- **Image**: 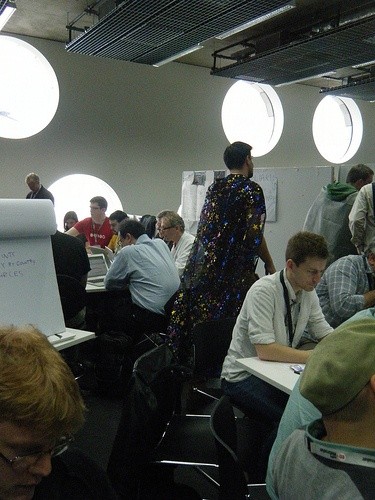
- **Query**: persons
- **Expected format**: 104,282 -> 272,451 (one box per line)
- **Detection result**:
104,218 -> 182,336
348,181 -> 375,255
64,211 -> 87,243
108,210 -> 130,253
219,233 -> 334,474
154,210 -> 177,251
0,323 -> 86,500
160,212 -> 197,278
298,242 -> 375,350
171,142 -> 276,380
65,196 -> 113,249
25,173 -> 55,205
266,308 -> 374,499
50,229 -> 91,329
303,164 -> 373,276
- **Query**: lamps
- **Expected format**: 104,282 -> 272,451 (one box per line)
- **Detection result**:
221,80 -> 284,158
312,94 -> 364,165
0,0 -> 17,30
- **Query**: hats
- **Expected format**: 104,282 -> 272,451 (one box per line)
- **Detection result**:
303,314 -> 375,415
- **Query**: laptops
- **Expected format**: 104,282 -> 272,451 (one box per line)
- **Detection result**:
86,246 -> 115,287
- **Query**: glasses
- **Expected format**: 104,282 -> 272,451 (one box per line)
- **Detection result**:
0,431 -> 74,474
159,225 -> 178,232
90,206 -> 101,211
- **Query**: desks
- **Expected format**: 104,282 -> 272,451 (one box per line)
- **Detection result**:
86,279 -> 108,329
48,328 -> 97,351
236,357 -> 305,395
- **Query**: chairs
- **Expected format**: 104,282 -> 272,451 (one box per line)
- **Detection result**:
150,387 -> 267,500
132,314 -> 165,373
